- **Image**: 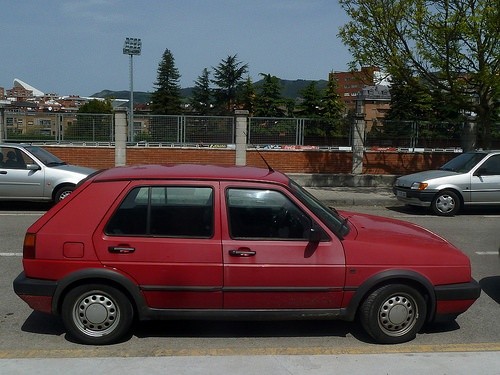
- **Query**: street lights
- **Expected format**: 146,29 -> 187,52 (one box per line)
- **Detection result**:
122,37 -> 143,143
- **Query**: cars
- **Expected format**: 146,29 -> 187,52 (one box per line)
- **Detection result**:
393,150 -> 499,216
14,132 -> 480,345
0,141 -> 100,204
